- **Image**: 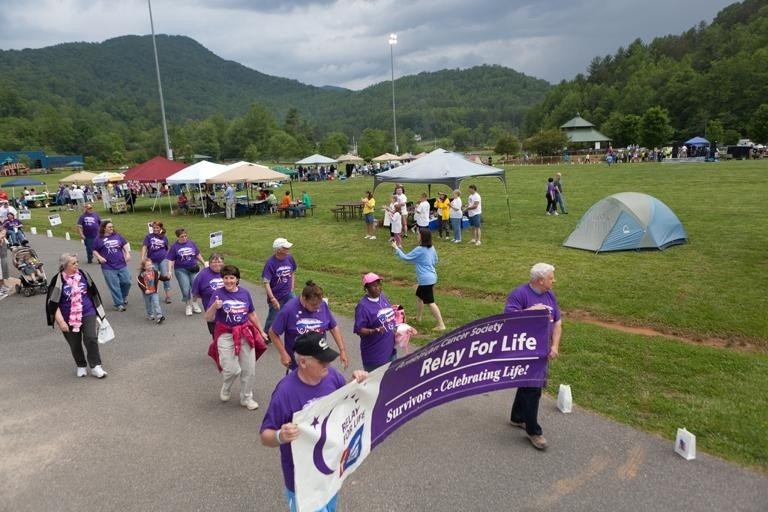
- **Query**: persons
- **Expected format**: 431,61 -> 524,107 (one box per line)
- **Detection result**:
263,237 -> 298,343
549,172 -> 568,214
361,185 -> 483,245
0,187 -> 50,299
267,279 -> 349,376
57,181 -> 146,211
544,177 -> 560,215
45,253 -> 116,378
298,160 -> 410,181
74,205 -> 270,410
585,153 -> 590,164
176,182 -> 313,221
606,143 -> 710,168
352,272 -> 419,373
390,229 -> 447,331
260,332 -> 368,512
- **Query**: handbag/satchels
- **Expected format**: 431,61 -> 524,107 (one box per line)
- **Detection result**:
557,383 -> 572,414
674,426 -> 698,461
94,315 -> 118,344
191,265 -> 200,274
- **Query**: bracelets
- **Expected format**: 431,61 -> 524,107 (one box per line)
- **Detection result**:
277,429 -> 283,445
270,296 -> 277,304
375,328 -> 381,336
369,328 -> 374,336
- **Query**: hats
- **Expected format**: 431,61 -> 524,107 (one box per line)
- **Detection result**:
273,237 -> 294,250
295,331 -> 339,364
360,271 -> 384,287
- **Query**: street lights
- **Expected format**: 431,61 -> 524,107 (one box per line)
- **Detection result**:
388,30 -> 398,153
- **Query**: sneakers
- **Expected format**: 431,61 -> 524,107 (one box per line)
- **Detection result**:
240,396 -> 259,411
114,297 -> 202,324
509,417 -> 548,450
219,384 -> 232,401
363,233 -> 482,251
77,364 -> 107,379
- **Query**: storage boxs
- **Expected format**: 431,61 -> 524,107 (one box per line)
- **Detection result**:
461,217 -> 472,230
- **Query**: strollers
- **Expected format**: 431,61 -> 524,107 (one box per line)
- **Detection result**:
4,240 -> 50,298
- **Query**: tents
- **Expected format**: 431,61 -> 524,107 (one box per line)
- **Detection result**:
562,192 -> 688,255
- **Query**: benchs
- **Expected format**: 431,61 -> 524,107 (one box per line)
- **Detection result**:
183,194 -> 442,230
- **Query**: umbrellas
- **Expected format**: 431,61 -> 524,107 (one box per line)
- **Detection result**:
687,137 -> 710,149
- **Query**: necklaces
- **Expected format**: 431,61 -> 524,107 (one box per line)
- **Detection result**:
505,263 -> 562,448
